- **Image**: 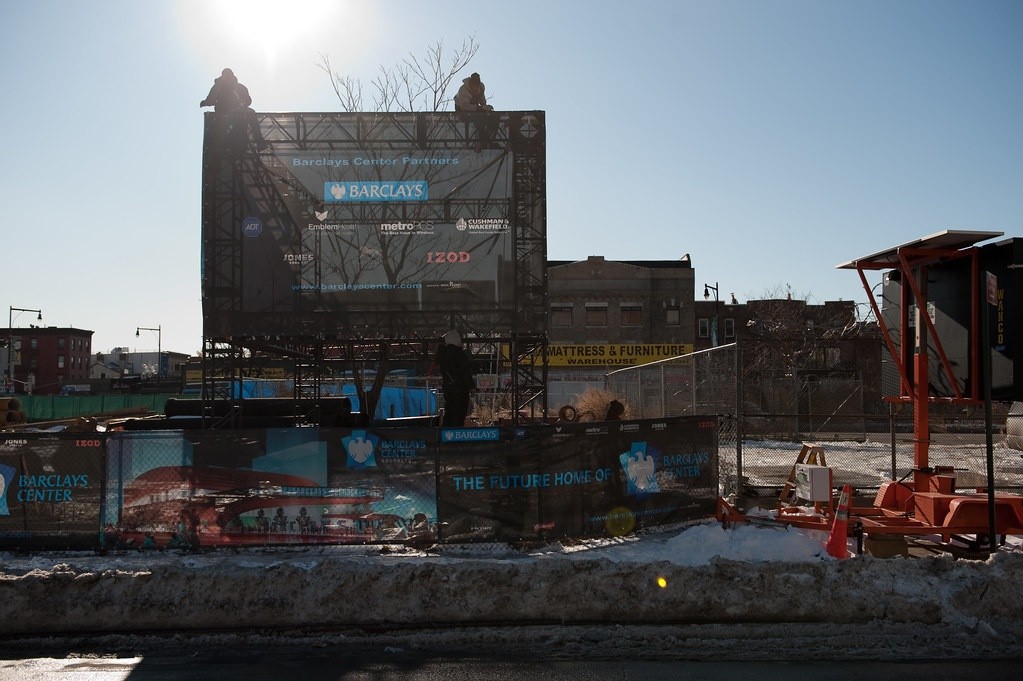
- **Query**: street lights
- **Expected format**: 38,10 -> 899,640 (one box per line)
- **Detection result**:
136,325 -> 161,380
8,306 -> 42,378
704,282 -> 720,346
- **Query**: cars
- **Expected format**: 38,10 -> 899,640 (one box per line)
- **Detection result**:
1005,402 -> 1023,452
741,401 -> 777,432
682,404 -> 751,433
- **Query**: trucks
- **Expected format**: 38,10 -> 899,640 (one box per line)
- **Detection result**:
62,384 -> 91,394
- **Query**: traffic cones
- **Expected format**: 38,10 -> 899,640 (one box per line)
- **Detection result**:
822,484 -> 852,558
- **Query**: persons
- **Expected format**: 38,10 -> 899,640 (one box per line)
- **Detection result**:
199,68 -> 270,152
453,73 -> 493,112
439,330 -> 476,426
605,401 -> 624,432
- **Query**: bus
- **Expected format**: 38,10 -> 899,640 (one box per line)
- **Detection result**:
134,382 -> 160,394
